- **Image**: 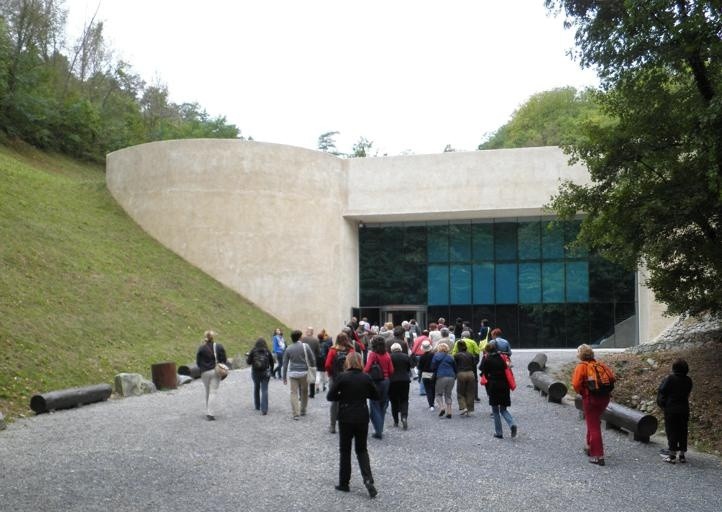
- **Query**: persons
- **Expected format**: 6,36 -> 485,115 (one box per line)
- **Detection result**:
656,357 -> 693,464
573,344 -> 616,466
326,351 -> 382,497
197,330 -> 228,419
246,314 -> 518,439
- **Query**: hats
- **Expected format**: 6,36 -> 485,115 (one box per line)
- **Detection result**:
421,340 -> 432,352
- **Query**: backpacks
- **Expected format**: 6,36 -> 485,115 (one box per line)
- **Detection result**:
254,353 -> 269,370
580,360 -> 614,394
332,351 -> 347,378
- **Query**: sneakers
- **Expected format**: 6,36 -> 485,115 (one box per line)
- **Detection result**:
335,485 -> 350,492
206,415 -> 215,420
663,455 -> 686,465
395,420 -> 407,429
430,407 -> 469,418
372,432 -> 381,439
255,408 -> 268,415
294,410 -> 306,420
494,424 -> 517,439
365,480 -> 377,498
584,449 -> 606,466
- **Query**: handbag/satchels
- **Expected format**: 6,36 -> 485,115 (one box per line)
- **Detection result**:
214,362 -> 229,380
504,366 -> 516,391
368,363 -> 385,381
422,371 -> 434,380
308,366 -> 317,383
